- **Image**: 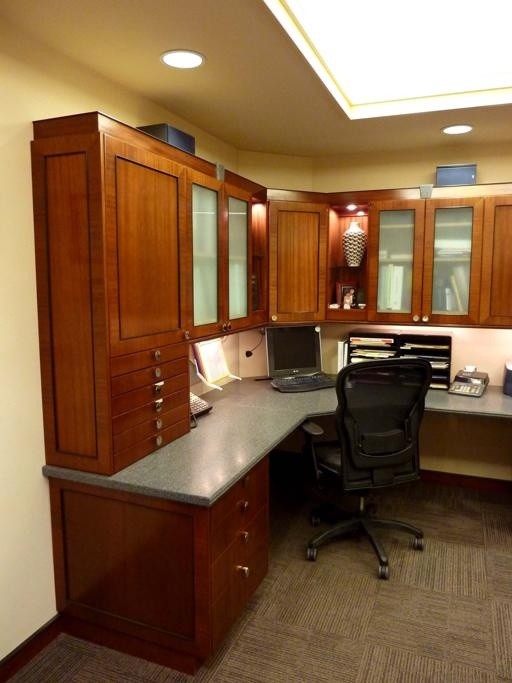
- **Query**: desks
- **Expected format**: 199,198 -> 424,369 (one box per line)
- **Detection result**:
42,381 -> 512,677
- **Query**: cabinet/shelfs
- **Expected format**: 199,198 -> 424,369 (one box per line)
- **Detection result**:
30,110 -> 512,477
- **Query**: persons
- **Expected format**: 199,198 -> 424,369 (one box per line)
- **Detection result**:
349,288 -> 355,296
344,292 -> 352,303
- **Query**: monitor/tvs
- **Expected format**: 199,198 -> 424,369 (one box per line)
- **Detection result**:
265,325 -> 322,378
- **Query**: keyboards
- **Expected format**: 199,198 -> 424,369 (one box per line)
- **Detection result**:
272,375 -> 337,393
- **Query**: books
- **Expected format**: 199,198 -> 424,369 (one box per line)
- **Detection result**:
350,336 -> 448,388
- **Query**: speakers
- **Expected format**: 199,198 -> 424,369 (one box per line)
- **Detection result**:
132,123 -> 196,155
436,164 -> 476,187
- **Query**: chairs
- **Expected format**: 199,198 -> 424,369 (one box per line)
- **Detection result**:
299,358 -> 433,579
